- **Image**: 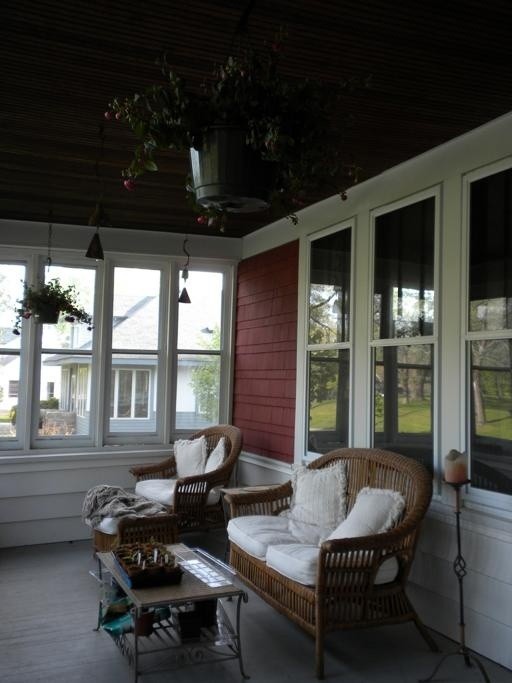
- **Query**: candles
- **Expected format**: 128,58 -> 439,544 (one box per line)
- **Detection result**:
444,450 -> 469,482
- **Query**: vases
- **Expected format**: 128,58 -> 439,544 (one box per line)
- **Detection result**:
33,310 -> 60,324
190,126 -> 272,213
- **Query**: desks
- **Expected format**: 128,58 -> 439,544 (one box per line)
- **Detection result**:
93,543 -> 251,682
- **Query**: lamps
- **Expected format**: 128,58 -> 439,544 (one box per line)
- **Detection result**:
178,239 -> 191,304
84,218 -> 104,261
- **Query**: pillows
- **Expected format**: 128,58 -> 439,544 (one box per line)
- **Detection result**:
288,463 -> 347,544
205,436 -> 227,474
173,435 -> 209,478
318,488 -> 406,557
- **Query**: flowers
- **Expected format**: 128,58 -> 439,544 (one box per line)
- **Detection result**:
12,278 -> 95,335
102,42 -> 356,228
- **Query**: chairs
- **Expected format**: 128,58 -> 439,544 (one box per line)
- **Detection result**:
88,514 -> 179,585
225,447 -> 438,677
129,425 -> 242,532
